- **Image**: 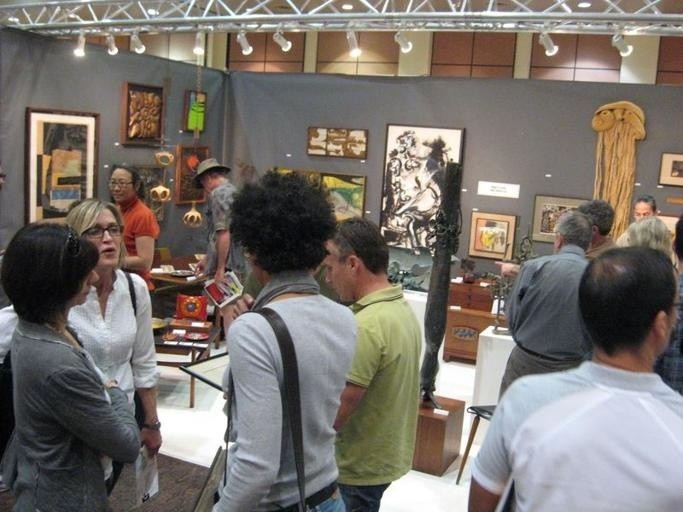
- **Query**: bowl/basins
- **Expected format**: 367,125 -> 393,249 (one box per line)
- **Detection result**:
152,318 -> 168,336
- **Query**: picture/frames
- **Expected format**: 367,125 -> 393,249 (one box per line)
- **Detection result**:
184,89 -> 208,133
659,153 -> 683,186
307,127 -> 368,158
176,143 -> 208,206
467,211 -> 517,262
24,105 -> 101,230
532,194 -> 590,243
119,79 -> 163,148
378,123 -> 465,252
131,166 -> 169,223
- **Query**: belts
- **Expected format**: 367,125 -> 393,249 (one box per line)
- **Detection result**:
277,480 -> 338,512
517,342 -> 558,362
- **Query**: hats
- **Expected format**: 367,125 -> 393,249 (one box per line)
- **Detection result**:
192,158 -> 230,178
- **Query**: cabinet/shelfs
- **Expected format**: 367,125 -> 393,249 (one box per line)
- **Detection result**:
443,306 -> 507,363
472,326 -> 515,408
447,277 -> 495,313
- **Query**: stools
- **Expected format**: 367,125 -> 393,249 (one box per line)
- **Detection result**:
456,403 -> 497,483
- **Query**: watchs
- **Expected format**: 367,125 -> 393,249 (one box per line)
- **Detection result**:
142,422 -> 161,429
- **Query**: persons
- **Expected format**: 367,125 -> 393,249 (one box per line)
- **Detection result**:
0,198 -> 162,512
191,157 -> 250,339
0,222 -> 141,512
499,210 -> 593,406
633,194 -> 656,219
231,256 -> 351,319
109,164 -> 160,296
628,214 -> 673,263
654,214 -> 683,399
495,199 -> 620,278
325,216 -> 421,512
468,247 -> 683,511
212,168 -> 359,512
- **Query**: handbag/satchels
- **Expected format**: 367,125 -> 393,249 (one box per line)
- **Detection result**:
0,349 -> 15,463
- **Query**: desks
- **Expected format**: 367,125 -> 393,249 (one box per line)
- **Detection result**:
148,254 -> 225,349
155,321 -> 221,407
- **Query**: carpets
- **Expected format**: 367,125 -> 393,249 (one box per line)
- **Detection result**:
1,454 -> 210,511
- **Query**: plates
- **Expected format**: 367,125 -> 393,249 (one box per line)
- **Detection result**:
170,270 -> 193,278
150,274 -> 168,276
185,332 -> 209,342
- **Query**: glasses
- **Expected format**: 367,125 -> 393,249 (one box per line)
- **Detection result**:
85,224 -> 124,237
672,296 -> 683,310
108,181 -> 134,187
58,224 -> 80,271
